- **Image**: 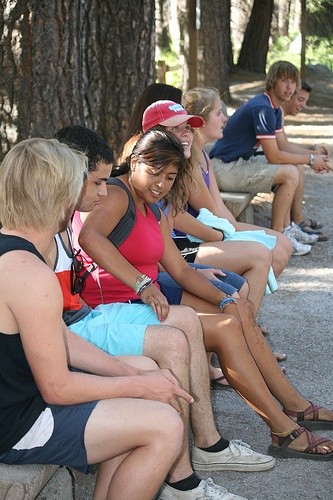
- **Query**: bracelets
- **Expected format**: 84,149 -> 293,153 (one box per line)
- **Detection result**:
308,154 -> 315,167
311,143 -> 316,150
132,274 -> 153,295
218,295 -> 237,312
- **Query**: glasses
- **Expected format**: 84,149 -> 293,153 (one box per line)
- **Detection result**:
69,248 -> 85,296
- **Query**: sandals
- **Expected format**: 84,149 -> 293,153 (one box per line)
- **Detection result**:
283,401 -> 333,427
295,223 -> 330,241
268,428 -> 333,460
302,218 -> 322,229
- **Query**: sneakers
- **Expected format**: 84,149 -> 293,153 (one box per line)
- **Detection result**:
279,221 -> 319,244
192,440 -> 277,472
158,477 -> 250,499
278,232 -> 311,256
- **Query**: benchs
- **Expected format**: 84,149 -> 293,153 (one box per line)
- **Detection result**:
0,463 -> 77,500
220,190 -> 256,226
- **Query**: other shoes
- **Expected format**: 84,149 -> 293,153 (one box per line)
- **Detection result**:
209,372 -> 238,389
274,352 -> 287,361
259,324 -> 270,336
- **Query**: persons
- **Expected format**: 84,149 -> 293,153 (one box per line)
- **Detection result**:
64,130 -> 333,463
20,124 -> 276,500
249,78 -> 329,241
115,83 -> 182,170
205,60 -> 333,256
103,166 -> 256,390
122,100 -> 288,361
182,83 -> 293,329
0,137 -> 194,500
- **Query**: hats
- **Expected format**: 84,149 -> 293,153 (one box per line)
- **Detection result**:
142,100 -> 204,133
